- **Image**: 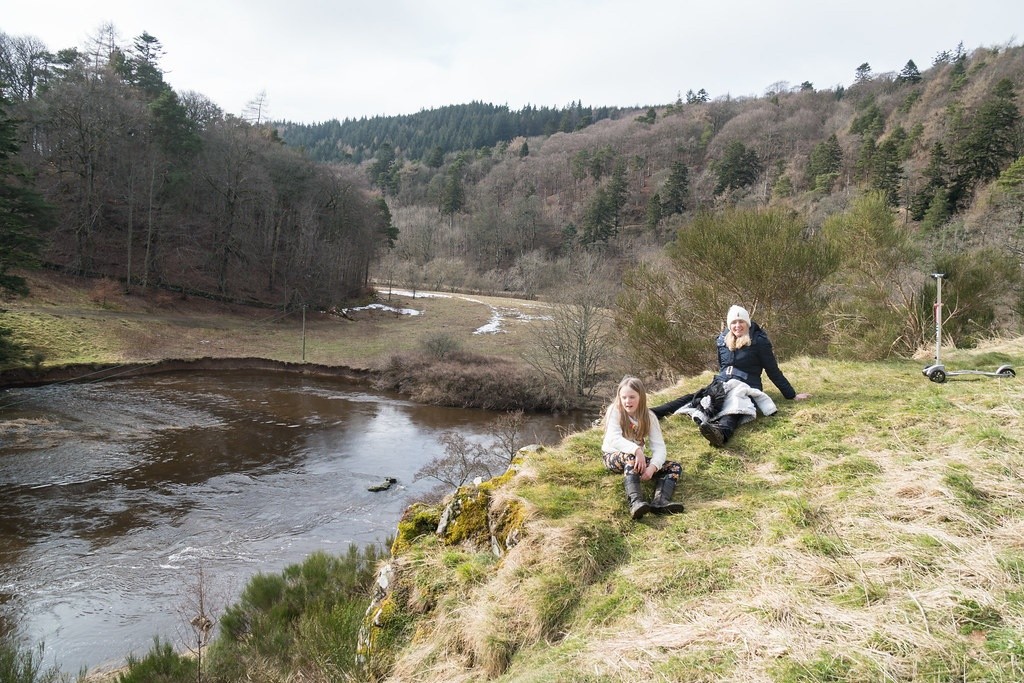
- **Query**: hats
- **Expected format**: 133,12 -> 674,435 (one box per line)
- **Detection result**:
727,304 -> 751,331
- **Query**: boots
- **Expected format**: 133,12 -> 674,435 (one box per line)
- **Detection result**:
622,475 -> 649,520
649,478 -> 684,515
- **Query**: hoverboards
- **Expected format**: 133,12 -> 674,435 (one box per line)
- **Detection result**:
921,273 -> 1016,383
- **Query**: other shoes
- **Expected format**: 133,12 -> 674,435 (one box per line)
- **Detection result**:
699,421 -> 725,448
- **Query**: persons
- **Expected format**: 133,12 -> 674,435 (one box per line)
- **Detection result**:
601,377 -> 684,521
648,304 -> 814,448
